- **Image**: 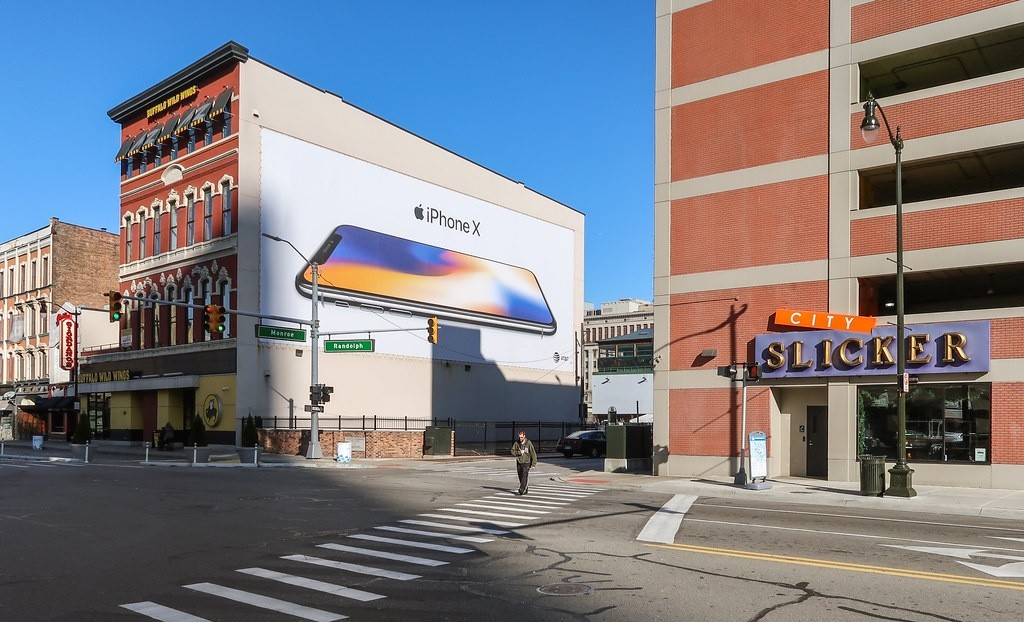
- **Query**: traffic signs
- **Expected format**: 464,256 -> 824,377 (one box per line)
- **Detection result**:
310,405 -> 324,413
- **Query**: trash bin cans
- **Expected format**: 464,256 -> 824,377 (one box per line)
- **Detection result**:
335,443 -> 352,462
32,435 -> 44,450
857,455 -> 887,496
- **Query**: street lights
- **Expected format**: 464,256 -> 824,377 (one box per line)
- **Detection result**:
38,300 -> 83,436
859,92 -> 919,500
260,231 -> 324,459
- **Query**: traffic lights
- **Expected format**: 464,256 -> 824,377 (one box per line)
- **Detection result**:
746,364 -> 762,379
213,305 -> 226,333
724,365 -> 737,376
309,386 -> 320,401
321,386 -> 333,403
427,317 -> 437,345
202,304 -> 213,333
109,289 -> 122,323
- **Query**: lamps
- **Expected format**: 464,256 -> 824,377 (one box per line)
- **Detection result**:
701,349 -> 717,357
264,370 -> 270,377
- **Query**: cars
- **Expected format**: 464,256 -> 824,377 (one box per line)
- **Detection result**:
554,429 -> 606,459
863,424 -> 977,461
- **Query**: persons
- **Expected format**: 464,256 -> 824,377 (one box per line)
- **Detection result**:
158,422 -> 175,451
511,431 -> 538,495
